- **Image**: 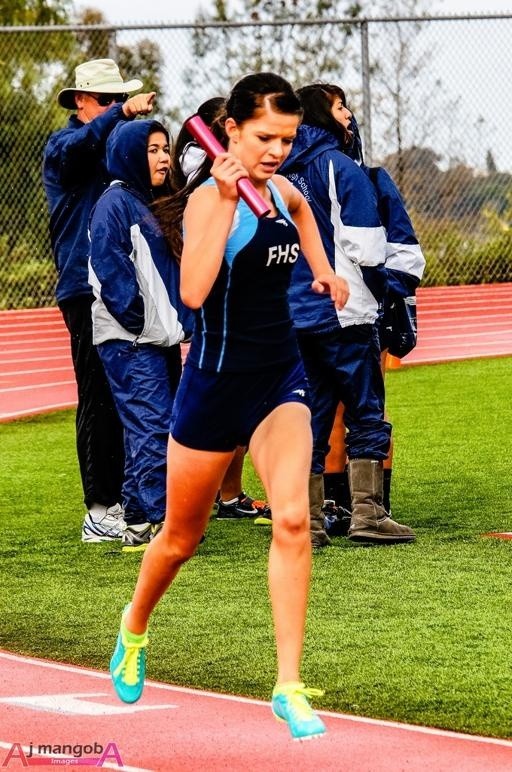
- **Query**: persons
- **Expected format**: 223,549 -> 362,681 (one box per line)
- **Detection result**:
41,55 -> 430,547
106,72 -> 351,744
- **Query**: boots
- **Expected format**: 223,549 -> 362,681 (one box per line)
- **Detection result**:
309,457 -> 418,550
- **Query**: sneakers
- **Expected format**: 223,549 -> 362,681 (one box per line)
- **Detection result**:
271,684 -> 326,741
121,524 -> 161,553
108,603 -> 149,703
215,494 -> 267,518
79,506 -> 124,540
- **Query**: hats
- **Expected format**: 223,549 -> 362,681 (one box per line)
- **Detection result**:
58,59 -> 144,103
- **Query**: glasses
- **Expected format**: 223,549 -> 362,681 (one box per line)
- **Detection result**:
82,91 -> 128,106
